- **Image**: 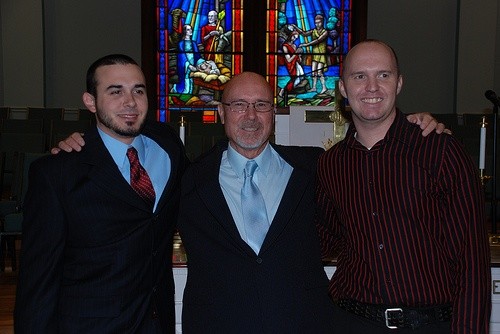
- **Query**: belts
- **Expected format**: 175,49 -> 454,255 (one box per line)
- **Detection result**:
333,293 -> 453,330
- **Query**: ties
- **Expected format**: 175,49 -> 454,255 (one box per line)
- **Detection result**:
241,160 -> 271,257
126,147 -> 156,214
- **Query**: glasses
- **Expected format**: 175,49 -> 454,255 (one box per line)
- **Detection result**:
222,100 -> 275,113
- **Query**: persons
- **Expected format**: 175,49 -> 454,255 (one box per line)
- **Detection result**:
49,72 -> 452,334
316,39 -> 491,334
13,54 -> 190,334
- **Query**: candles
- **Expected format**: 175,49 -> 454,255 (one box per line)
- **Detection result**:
479,116 -> 488,170
179,115 -> 184,146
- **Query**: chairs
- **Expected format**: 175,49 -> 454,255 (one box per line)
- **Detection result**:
0,107 -> 97,274
156,112 -> 226,162
435,113 -> 500,221
